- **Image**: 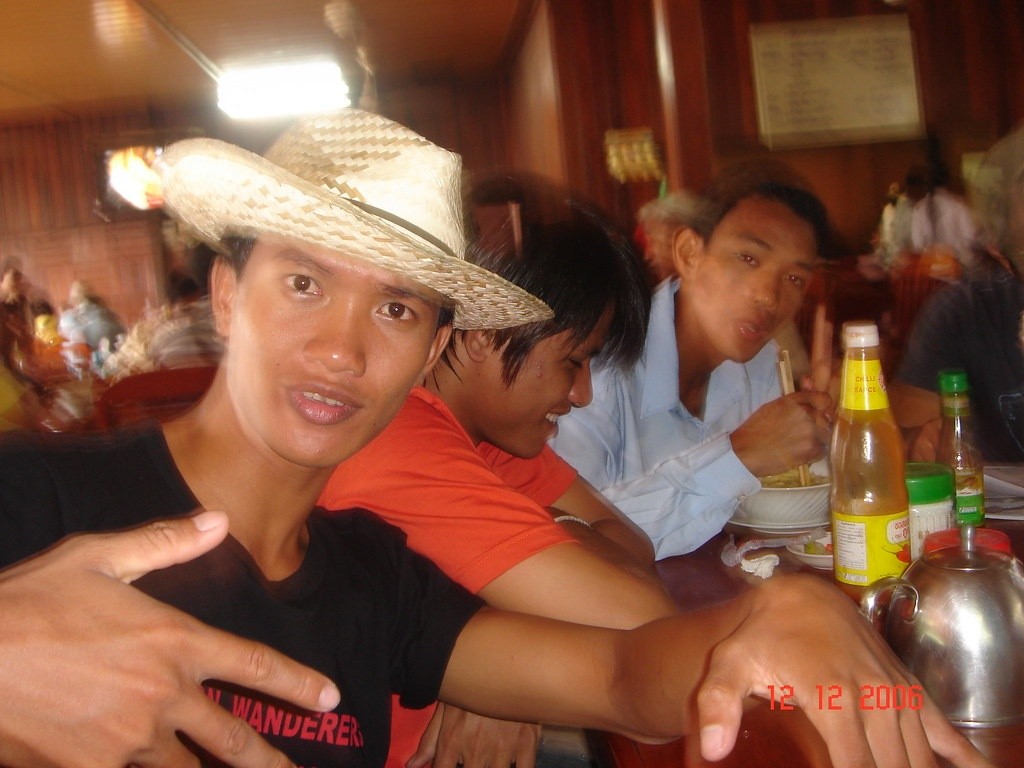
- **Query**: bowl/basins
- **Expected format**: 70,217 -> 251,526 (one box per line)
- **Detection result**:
739,457 -> 833,524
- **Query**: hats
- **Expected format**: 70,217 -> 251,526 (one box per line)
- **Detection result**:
159,108 -> 558,330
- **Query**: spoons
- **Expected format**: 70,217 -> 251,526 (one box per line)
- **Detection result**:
986,505 -> 1024,513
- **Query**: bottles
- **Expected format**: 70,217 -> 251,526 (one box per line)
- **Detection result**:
937,372 -> 985,528
830,322 -> 911,605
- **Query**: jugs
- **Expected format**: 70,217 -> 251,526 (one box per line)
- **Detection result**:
860,527 -> 1024,728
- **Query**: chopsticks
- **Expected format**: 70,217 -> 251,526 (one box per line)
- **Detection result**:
779,350 -> 812,486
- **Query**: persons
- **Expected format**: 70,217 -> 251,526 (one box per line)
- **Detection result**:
0,135 -> 1024,768
0,109 -> 1001,768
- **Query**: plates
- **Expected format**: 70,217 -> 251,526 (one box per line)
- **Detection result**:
787,532 -> 834,570
728,517 -> 831,535
984,472 -> 1024,521
